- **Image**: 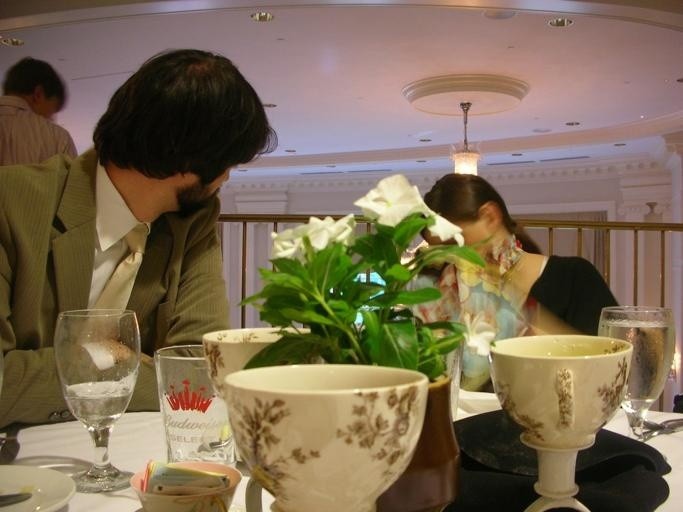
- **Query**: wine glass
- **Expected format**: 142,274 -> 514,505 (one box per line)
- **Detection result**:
599,306 -> 676,443
487,332 -> 633,512
52,307 -> 142,494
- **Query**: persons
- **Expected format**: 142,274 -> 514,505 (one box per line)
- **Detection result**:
0,50 -> 279,441
1,53 -> 79,165
417,171 -> 656,400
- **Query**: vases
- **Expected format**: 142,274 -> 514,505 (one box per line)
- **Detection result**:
375,375 -> 464,511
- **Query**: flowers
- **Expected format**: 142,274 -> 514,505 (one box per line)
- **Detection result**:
231,175 -> 493,383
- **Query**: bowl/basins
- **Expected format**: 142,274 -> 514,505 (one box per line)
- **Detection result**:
130,460 -> 242,512
202,327 -> 311,398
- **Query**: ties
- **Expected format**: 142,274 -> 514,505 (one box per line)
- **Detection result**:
85,224 -> 147,317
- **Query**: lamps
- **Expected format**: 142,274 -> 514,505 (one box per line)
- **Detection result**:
451,101 -> 481,175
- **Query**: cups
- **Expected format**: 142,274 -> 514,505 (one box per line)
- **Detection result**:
224,362 -> 428,512
154,344 -> 240,464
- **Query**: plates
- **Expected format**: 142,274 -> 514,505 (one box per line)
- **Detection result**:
0,465 -> 77,510
